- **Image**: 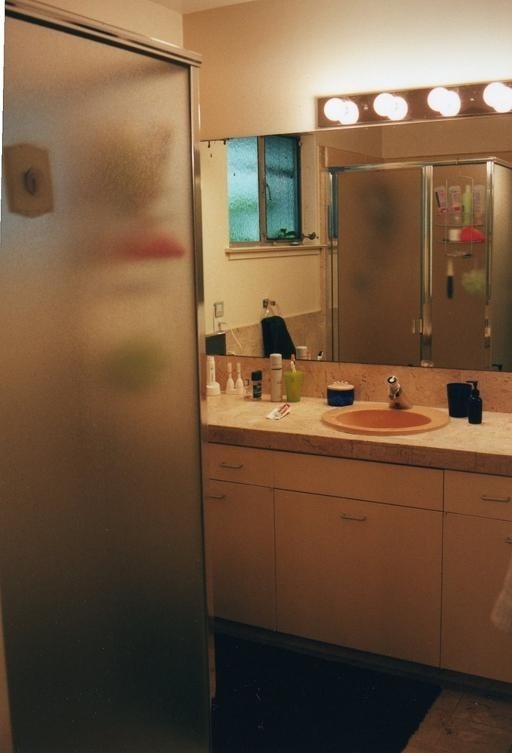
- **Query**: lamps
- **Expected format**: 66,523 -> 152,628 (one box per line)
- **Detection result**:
374,94 -> 407,121
324,97 -> 359,125
428,87 -> 460,117
484,82 -> 512,113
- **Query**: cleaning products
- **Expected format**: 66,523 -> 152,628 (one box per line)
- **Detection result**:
434,186 -> 449,223
449,185 -> 462,224
462,185 -> 472,224
473,184 -> 486,224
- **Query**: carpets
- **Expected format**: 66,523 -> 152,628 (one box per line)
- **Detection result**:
209,634 -> 443,753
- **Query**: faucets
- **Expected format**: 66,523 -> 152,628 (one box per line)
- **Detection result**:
387,375 -> 413,410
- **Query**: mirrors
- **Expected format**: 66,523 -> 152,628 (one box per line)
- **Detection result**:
199,114 -> 512,372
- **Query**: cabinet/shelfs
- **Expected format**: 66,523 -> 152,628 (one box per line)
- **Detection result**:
328,156 -> 512,372
205,442 -> 512,687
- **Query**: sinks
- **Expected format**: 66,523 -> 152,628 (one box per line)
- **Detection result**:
321,404 -> 450,436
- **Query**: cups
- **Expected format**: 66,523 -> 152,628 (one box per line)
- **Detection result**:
447,383 -> 471,418
283,371 -> 304,402
448,229 -> 461,241
